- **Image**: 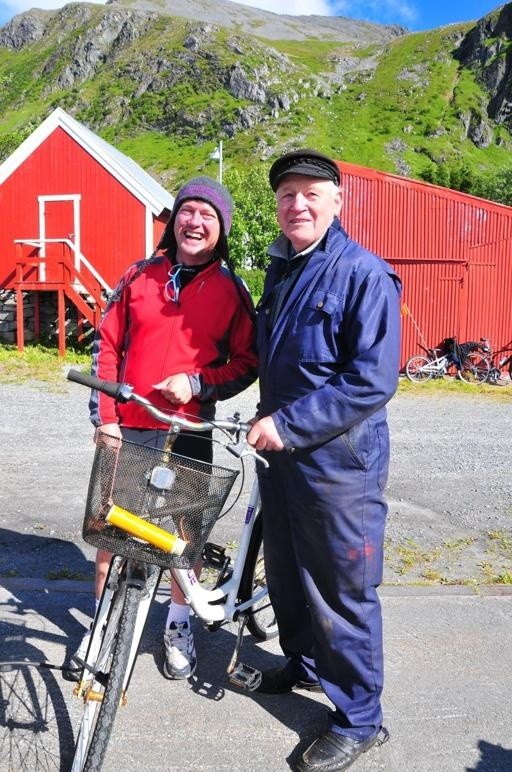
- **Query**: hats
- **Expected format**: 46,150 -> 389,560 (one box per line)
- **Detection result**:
269,150 -> 340,193
158,175 -> 234,260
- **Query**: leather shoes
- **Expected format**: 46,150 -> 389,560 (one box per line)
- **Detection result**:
257,659 -> 325,695
295,730 -> 378,772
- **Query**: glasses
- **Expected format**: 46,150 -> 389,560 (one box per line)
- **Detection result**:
165,263 -> 183,303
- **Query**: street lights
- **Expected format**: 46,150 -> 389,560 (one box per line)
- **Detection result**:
211,140 -> 224,185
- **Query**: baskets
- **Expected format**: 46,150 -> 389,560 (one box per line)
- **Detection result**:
82,432 -> 242,570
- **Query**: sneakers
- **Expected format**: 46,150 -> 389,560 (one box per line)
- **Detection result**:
163,620 -> 198,680
66,621 -> 109,683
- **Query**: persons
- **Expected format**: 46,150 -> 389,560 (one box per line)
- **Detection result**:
245,149 -> 402,772
66,177 -> 256,686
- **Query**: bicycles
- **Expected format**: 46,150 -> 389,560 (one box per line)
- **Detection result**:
458,337 -> 512,387
405,335 -> 491,385
66,366 -> 281,771
